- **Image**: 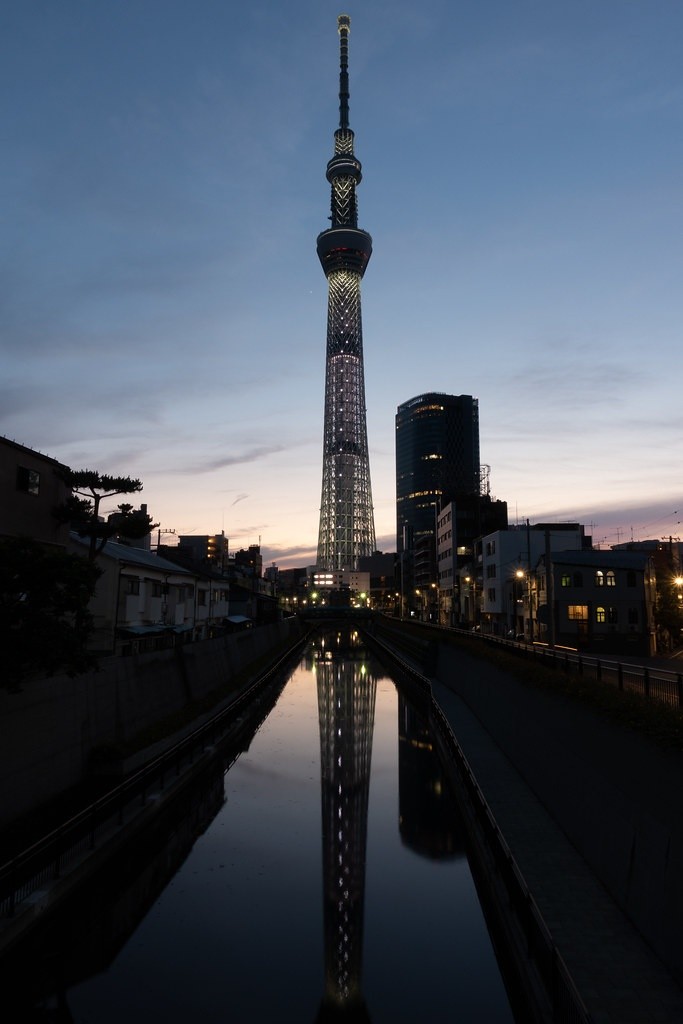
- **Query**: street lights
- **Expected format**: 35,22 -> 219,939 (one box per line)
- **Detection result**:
516,569 -> 534,644
465,577 -> 476,631
416,590 -> 423,622
432,584 -> 442,625
